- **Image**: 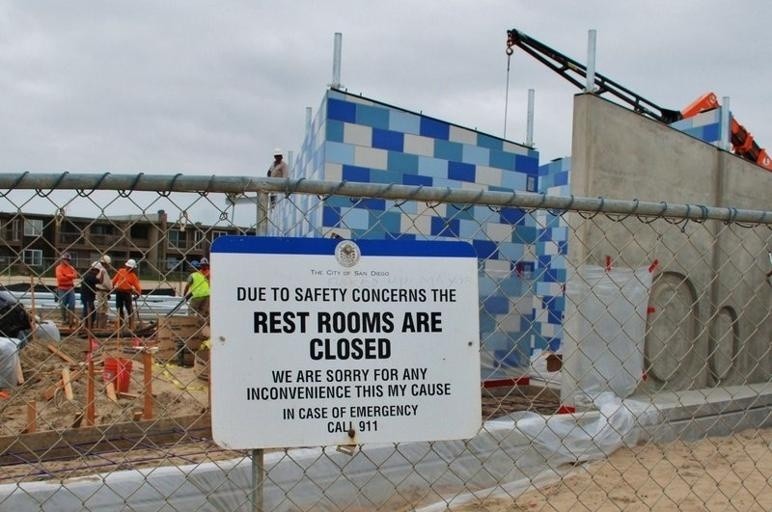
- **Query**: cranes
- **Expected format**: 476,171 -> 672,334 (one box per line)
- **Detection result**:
504,27 -> 772,169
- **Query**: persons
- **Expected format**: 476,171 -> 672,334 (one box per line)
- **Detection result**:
80,261 -> 106,329
183,260 -> 210,318
200,257 -> 210,287
56,252 -> 79,326
266,149 -> 288,208
92,254 -> 112,329
111,258 -> 141,330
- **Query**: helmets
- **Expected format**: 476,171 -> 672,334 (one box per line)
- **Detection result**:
63,253 -> 71,259
192,258 -> 209,267
125,259 -> 137,268
92,261 -> 101,269
101,255 -> 111,263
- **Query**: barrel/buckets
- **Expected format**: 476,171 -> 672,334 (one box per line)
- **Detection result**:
103,355 -> 132,394
194,351 -> 210,380
